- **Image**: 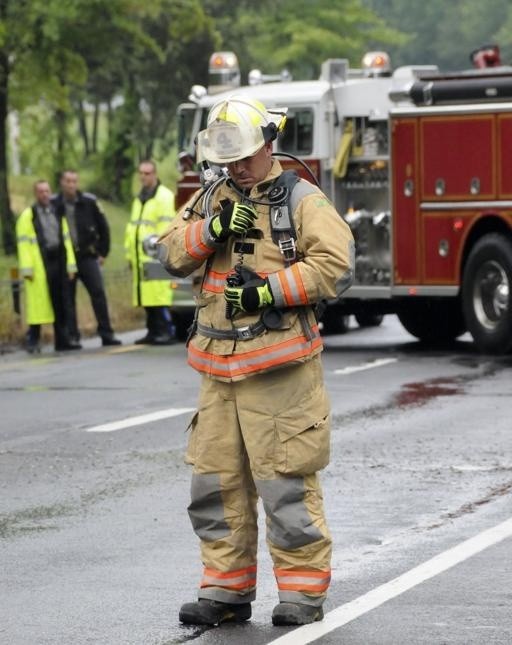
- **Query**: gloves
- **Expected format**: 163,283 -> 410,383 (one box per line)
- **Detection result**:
207,201 -> 259,244
222,264 -> 274,315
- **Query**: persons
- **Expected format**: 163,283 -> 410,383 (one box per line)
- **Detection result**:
15,169 -> 121,355
124,161 -> 176,345
156,97 -> 355,624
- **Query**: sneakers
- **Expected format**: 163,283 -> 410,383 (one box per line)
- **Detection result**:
177,598 -> 253,627
24,329 -> 177,358
270,601 -> 325,626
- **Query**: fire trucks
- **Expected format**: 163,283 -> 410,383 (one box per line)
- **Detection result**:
143,50 -> 511,349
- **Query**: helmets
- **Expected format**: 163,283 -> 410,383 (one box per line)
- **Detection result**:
200,92 -> 289,164
